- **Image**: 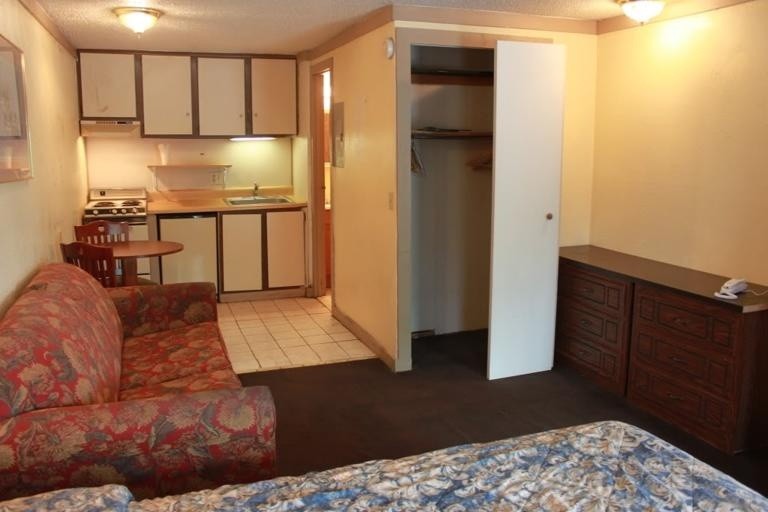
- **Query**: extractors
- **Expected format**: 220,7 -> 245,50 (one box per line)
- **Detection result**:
79,119 -> 140,138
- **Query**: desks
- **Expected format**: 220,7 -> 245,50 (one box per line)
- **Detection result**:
79,240 -> 184,285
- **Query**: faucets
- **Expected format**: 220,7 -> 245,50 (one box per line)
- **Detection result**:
252,183 -> 260,197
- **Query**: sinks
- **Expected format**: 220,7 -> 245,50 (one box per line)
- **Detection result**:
222,195 -> 293,206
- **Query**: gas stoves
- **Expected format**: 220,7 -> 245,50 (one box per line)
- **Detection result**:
84,199 -> 147,217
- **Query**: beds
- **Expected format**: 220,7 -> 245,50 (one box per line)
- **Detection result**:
0,417 -> 767,511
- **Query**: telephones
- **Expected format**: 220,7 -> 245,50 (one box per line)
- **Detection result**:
720,278 -> 748,295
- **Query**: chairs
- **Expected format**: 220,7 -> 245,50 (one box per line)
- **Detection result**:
74,220 -> 129,277
59,241 -> 117,287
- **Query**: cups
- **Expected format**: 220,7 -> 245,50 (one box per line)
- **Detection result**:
157,142 -> 170,166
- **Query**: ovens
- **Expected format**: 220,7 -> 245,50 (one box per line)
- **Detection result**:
83,218 -> 151,281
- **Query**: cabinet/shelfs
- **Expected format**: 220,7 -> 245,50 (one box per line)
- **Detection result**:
556,262 -> 632,404
158,216 -> 218,295
79,51 -> 137,119
613,0 -> 670,25
141,54 -> 194,135
628,282 -> 767,454
221,209 -> 305,292
197,56 -> 298,136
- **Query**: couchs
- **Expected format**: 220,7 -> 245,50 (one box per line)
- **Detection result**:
0,262 -> 279,502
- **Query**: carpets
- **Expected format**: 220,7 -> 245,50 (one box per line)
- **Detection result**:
216,296 -> 379,374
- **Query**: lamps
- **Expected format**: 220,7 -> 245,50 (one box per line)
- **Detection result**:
112,7 -> 161,33
